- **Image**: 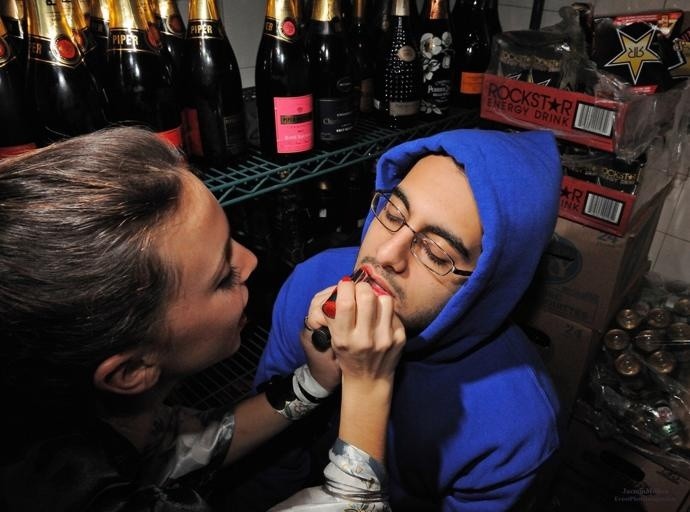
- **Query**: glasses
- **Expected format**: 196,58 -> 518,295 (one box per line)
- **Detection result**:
370,189 -> 473,276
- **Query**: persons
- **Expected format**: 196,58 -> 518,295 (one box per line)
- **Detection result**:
0,119 -> 407,512
246,128 -> 566,512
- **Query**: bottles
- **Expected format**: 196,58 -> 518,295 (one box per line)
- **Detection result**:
0,0 -> 488,171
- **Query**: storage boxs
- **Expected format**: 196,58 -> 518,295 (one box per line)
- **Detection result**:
478,71 -> 689,151
537,400 -> 688,511
478,121 -> 680,419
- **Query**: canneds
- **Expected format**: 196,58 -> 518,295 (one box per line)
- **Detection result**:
586,281 -> 690,460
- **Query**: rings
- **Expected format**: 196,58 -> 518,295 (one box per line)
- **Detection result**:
303,315 -> 314,332
